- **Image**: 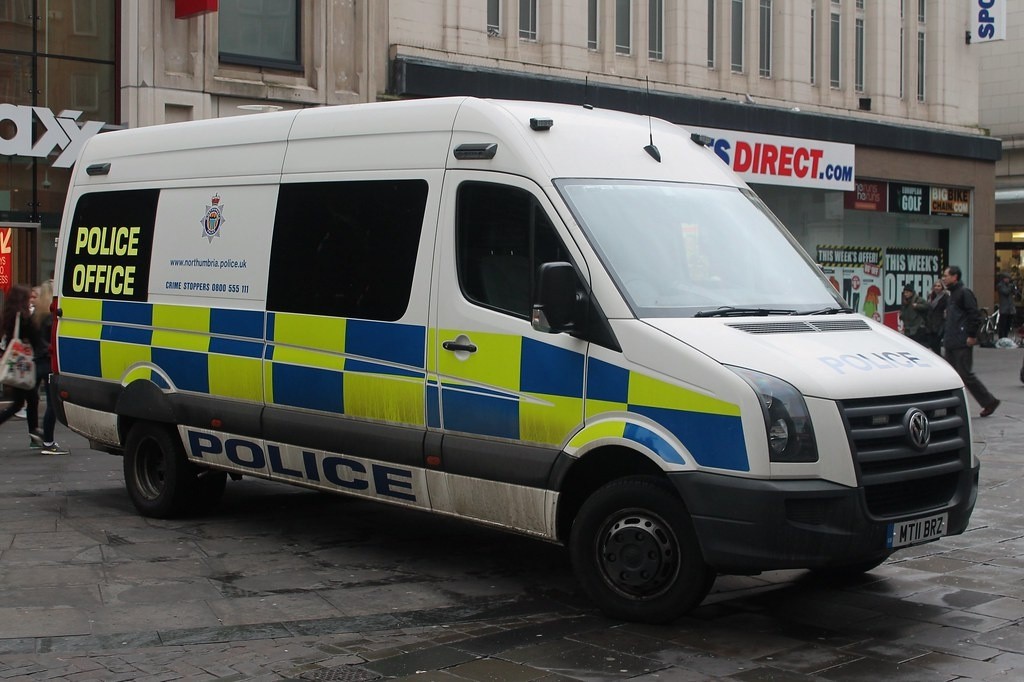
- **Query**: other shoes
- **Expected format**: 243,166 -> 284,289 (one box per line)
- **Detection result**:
30,443 -> 43,449
980,399 -> 1000,417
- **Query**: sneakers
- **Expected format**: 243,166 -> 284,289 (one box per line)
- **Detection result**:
41,442 -> 69,454
28,429 -> 43,439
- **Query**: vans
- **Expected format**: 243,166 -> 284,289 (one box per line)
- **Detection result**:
47,96 -> 983,626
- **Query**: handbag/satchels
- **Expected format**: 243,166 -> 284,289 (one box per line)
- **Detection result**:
0,312 -> 37,390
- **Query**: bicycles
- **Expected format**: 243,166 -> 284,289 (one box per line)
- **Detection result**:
979,303 -> 1015,344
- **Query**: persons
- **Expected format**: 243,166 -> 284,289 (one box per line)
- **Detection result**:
927,278 -> 950,354
997,273 -> 1017,340
0,278 -> 70,454
899,283 -> 927,347
942,265 -> 1001,417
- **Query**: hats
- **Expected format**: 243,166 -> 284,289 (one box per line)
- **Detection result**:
904,284 -> 914,292
1002,273 -> 1009,277
